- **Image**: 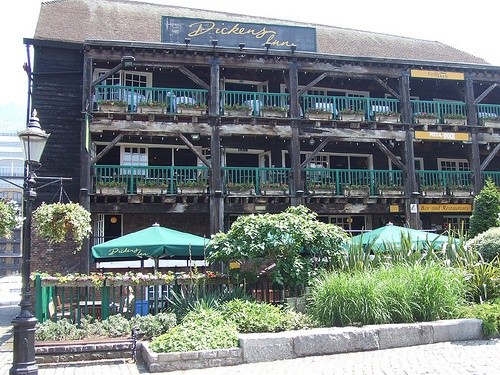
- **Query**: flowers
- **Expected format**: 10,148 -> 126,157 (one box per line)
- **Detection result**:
98,100 -> 500,123
413,112 -> 441,120
31,270 -> 228,288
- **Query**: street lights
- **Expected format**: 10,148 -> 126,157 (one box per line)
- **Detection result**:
9,107 -> 49,374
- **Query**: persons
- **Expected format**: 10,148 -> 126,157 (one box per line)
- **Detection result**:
117,286 -> 134,316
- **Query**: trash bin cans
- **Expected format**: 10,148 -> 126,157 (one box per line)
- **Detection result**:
133,300 -> 149,317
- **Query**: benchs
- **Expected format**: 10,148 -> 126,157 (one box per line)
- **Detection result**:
48,296 -> 134,323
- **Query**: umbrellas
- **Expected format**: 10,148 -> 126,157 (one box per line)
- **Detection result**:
341,222 -> 465,255
92,222 -> 214,313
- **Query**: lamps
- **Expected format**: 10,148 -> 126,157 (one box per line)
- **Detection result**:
290,45 -> 296,53
192,133 -> 201,139
185,38 -> 192,47
264,43 -> 271,52
239,43 -> 245,50
211,40 -> 219,48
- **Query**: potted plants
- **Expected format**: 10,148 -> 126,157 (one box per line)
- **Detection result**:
31,201 -> 94,254
96,179 -> 475,198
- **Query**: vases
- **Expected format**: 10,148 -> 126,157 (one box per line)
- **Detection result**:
106,280 -> 135,286
41,280 -> 95,288
140,279 -> 166,287
176,279 -> 199,285
206,278 -> 228,286
415,118 -> 439,126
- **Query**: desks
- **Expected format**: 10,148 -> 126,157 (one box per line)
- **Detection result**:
79,300 -> 101,317
